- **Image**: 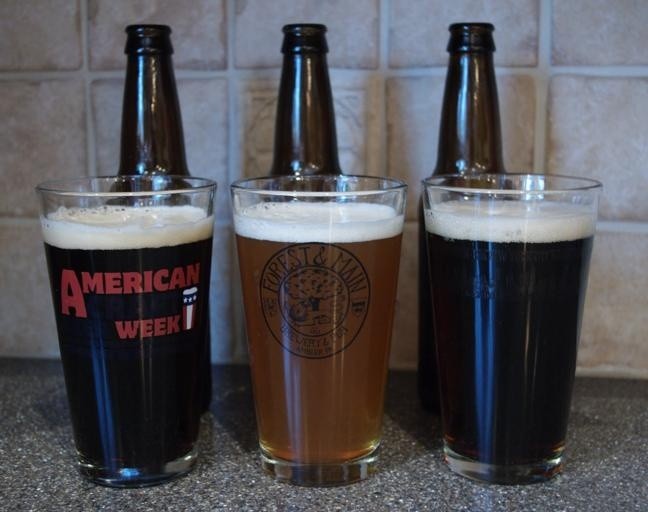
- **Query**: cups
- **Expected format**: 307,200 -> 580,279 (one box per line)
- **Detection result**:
36,175 -> 217,489
230,174 -> 409,487
420,173 -> 603,485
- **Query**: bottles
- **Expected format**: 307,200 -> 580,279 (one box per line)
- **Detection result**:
105,23 -> 196,208
264,23 -> 354,206
416,25 -> 519,414
